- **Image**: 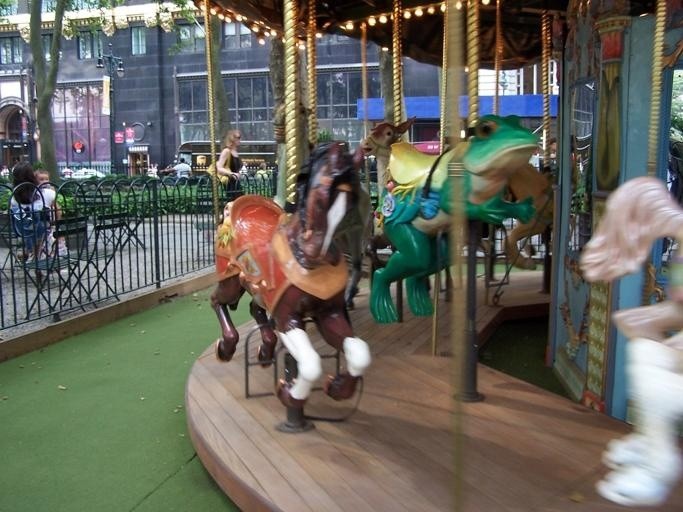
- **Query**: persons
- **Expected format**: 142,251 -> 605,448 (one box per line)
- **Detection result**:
548,137 -> 557,157
216,129 -> 247,201
256,162 -> 268,179
6,161 -> 56,262
163,158 -> 192,178
27,166 -> 63,225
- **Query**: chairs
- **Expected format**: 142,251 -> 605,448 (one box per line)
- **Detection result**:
194,197 -> 232,262
19,212 -> 129,323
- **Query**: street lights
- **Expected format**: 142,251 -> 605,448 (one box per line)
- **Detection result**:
95,43 -> 124,173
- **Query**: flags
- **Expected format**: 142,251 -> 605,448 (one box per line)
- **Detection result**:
101,74 -> 110,118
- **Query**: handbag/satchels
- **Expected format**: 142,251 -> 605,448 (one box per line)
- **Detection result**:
204,160 -> 232,186
